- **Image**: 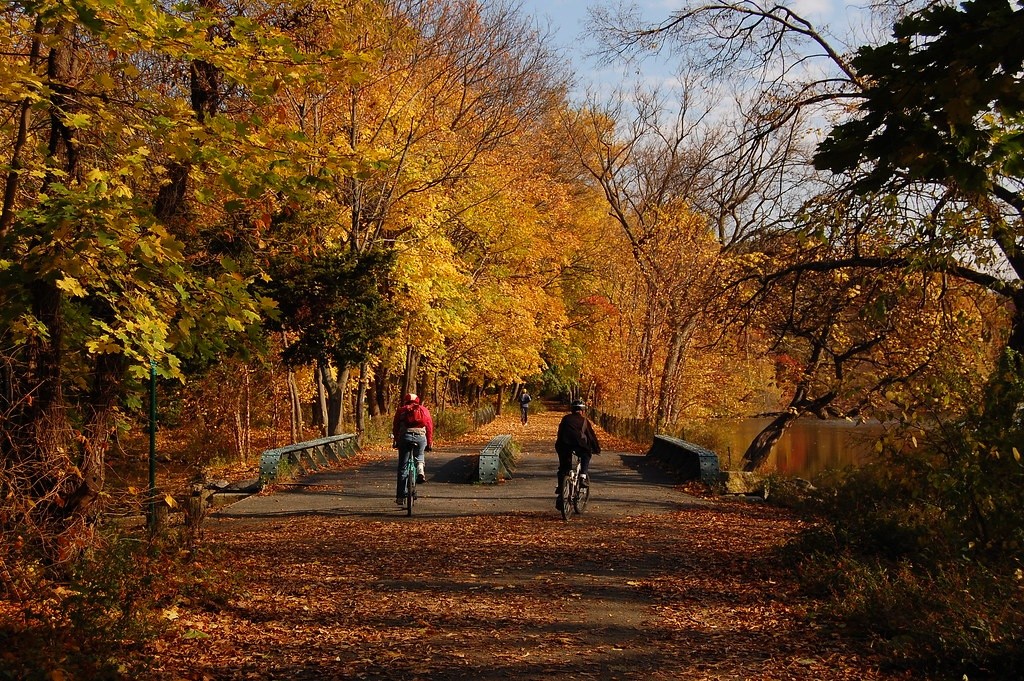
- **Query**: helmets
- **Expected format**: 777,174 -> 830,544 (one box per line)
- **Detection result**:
569,400 -> 586,411
404,393 -> 420,405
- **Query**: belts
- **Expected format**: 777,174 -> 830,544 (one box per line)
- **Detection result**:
406,432 -> 427,435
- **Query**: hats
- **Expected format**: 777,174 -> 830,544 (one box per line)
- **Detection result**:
522,389 -> 527,393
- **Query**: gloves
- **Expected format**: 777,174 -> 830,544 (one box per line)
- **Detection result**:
393,443 -> 398,450
424,445 -> 433,452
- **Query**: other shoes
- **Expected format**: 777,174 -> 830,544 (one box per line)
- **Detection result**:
415,471 -> 425,484
555,486 -> 567,494
397,498 -> 405,508
579,477 -> 590,488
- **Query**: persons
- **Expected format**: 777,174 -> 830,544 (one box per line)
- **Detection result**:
518,388 -> 531,426
555,401 -> 601,511
392,393 -> 432,505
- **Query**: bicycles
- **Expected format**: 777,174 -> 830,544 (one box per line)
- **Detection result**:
392,443 -> 432,517
557,450 -> 603,520
